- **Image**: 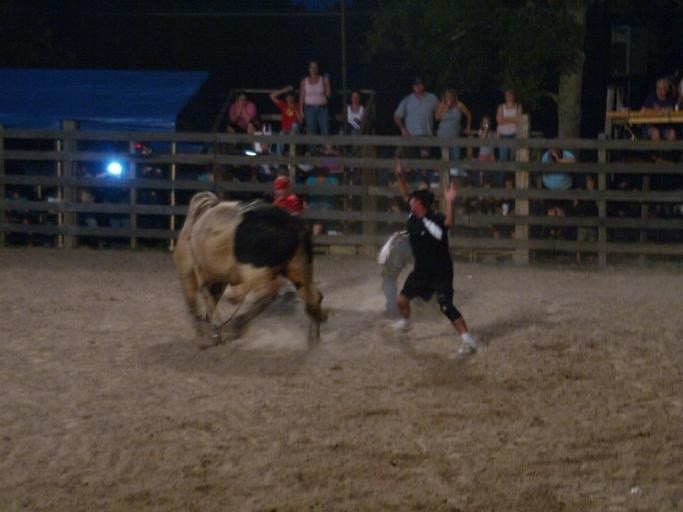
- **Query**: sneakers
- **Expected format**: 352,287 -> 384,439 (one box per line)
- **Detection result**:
458,339 -> 478,357
397,319 -> 414,334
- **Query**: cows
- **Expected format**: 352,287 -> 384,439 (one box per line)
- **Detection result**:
171,189 -> 329,346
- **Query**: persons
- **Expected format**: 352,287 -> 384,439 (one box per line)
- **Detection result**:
298,59 -> 333,152
269,174 -> 305,218
335,88 -> 368,172
296,145 -> 344,235
269,85 -> 302,170
132,141 -> 171,199
475,114 -> 495,186
392,155 -> 477,356
494,85 -> 521,189
434,87 -> 471,161
223,92 -> 259,153
392,76 -> 440,182
80,165 -> 113,226
639,75 -> 678,164
569,175 -> 599,263
536,137 -> 576,239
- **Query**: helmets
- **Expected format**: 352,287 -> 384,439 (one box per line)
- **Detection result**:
273,176 -> 292,190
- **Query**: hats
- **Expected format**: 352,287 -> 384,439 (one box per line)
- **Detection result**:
411,188 -> 436,203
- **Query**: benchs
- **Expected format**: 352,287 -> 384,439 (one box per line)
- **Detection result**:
213,86 -> 682,260
